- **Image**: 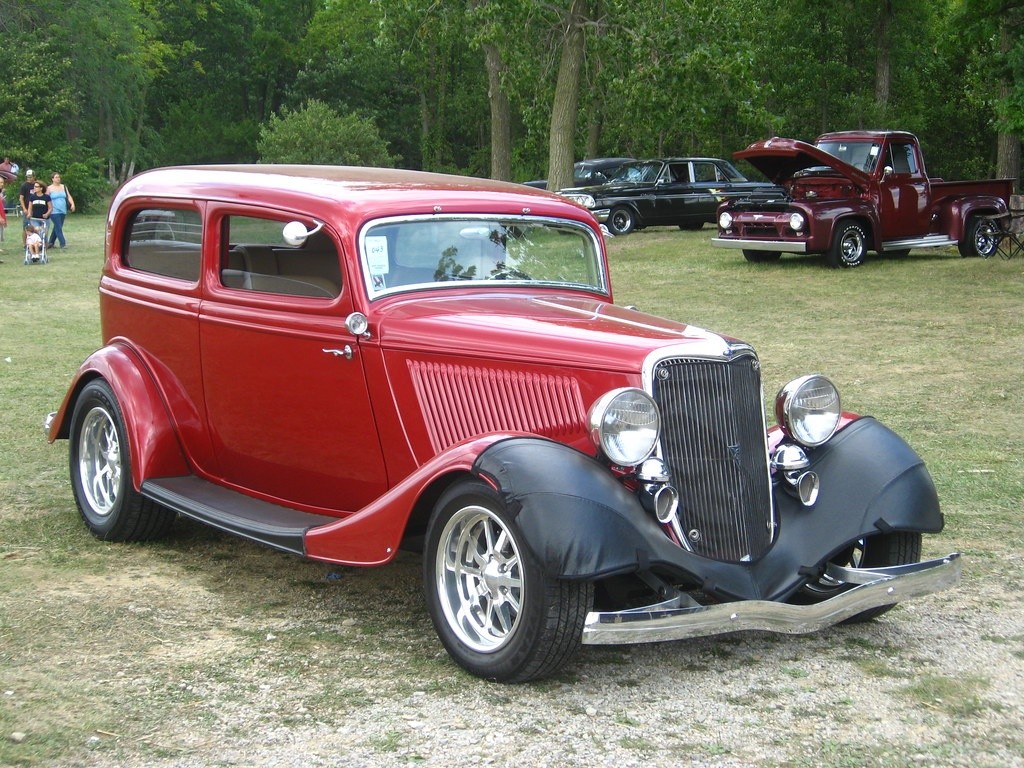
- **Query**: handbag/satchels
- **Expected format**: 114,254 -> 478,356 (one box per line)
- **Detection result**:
11,164 -> 19,175
64,184 -> 71,211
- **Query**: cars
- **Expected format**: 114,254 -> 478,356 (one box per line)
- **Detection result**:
520,157 -> 647,193
44,165 -> 963,684
555,156 -> 787,237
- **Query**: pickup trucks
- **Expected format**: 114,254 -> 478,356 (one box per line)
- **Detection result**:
710,128 -> 1017,271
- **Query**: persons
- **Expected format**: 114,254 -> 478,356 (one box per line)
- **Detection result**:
0,156 -> 53,263
45,171 -> 76,252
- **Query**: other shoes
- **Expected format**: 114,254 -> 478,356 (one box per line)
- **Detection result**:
32,255 -> 39,260
48,243 -> 57,248
0,260 -> 4,263
60,245 -> 67,249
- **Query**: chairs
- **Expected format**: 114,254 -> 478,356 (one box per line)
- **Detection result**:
975,194 -> 1024,261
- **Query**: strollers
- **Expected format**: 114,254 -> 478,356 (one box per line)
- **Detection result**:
24,217 -> 50,265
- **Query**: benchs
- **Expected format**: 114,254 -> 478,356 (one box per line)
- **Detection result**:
128,240 -> 343,299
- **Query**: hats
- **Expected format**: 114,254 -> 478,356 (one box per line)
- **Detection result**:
25,170 -> 35,176
